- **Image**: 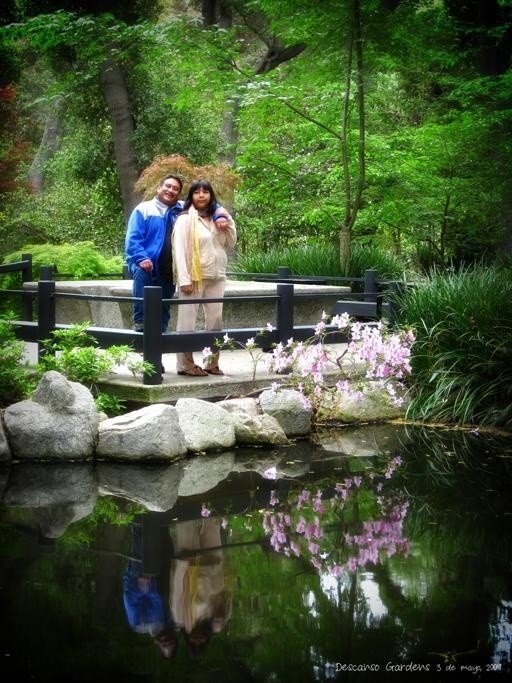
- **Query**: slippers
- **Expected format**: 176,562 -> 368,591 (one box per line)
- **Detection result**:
177,365 -> 224,376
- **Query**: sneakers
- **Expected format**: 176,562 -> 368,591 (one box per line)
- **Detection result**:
134,321 -> 144,332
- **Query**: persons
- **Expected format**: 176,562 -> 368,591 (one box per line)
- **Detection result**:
120,509 -> 232,658
166,516 -> 233,654
171,178 -> 239,376
125,173 -> 229,373
